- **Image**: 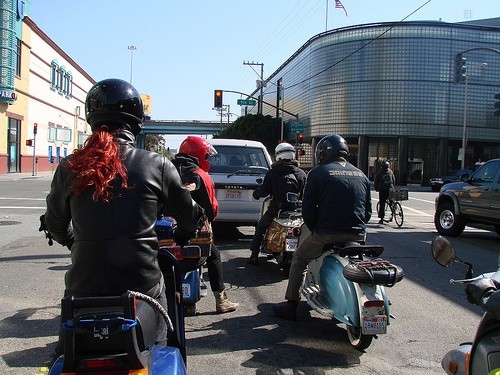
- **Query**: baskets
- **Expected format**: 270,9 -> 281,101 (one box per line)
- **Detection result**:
389,187 -> 408,201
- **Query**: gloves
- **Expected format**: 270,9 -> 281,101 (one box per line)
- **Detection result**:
466,277 -> 497,310
253,187 -> 260,200
174,227 -> 199,247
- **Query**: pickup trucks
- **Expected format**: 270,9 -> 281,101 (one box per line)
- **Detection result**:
429,168 -> 475,192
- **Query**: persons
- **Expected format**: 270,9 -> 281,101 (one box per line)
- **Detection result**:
272,134 -> 373,322
465,277 -> 500,321
44,79 -> 206,355
374,161 -> 395,224
170,135 -> 239,313
247,143 -> 306,265
183,175 -> 197,191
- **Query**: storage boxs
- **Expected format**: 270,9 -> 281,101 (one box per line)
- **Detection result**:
388,187 -> 408,201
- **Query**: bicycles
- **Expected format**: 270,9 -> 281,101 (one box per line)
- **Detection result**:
372,181 -> 404,227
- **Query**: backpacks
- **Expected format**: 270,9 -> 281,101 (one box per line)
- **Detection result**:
268,168 -> 304,209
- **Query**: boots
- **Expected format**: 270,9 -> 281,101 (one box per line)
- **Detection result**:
214,289 -> 236,314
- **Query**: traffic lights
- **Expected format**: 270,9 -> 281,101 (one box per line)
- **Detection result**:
214,90 -> 222,107
494,92 -> 500,117
34,123 -> 37,134
454,53 -> 467,82
298,134 -> 303,144
26,140 -> 32,145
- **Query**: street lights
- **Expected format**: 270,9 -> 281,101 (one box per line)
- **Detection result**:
128,45 -> 136,84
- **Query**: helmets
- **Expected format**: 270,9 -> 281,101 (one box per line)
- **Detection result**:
315,134 -> 349,165
85,78 -> 143,129
381,160 -> 390,169
179,136 -> 214,172
275,143 -> 294,161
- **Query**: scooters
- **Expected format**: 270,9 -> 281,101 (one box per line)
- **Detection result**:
430,234 -> 500,375
38,213 -> 202,375
253,177 -> 303,275
160,209 -> 219,318
285,191 -> 406,348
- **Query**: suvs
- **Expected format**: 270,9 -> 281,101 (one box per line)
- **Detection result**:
200,138 -> 273,232
434,159 -> 500,238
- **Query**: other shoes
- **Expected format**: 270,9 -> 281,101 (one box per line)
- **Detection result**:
247,255 -> 258,264
272,301 -> 299,321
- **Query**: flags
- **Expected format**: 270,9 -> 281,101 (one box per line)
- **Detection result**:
335,0 -> 348,16
